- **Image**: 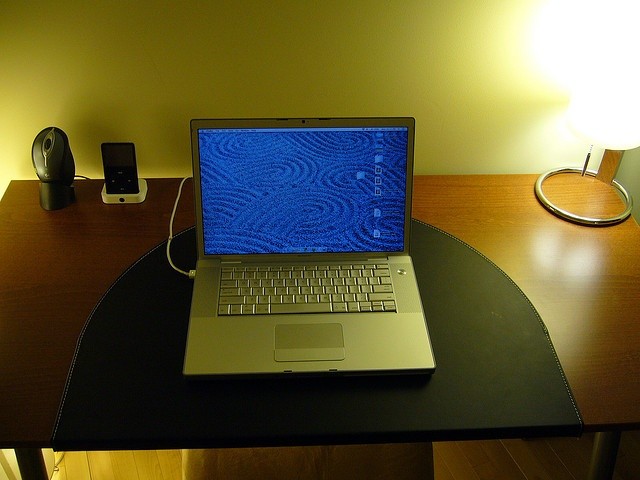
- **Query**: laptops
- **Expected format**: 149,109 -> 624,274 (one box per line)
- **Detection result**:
181,117 -> 438,382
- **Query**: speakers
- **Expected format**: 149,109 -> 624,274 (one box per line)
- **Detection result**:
31,124 -> 75,211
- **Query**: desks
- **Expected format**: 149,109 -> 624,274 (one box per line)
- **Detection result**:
1,171 -> 639,480
